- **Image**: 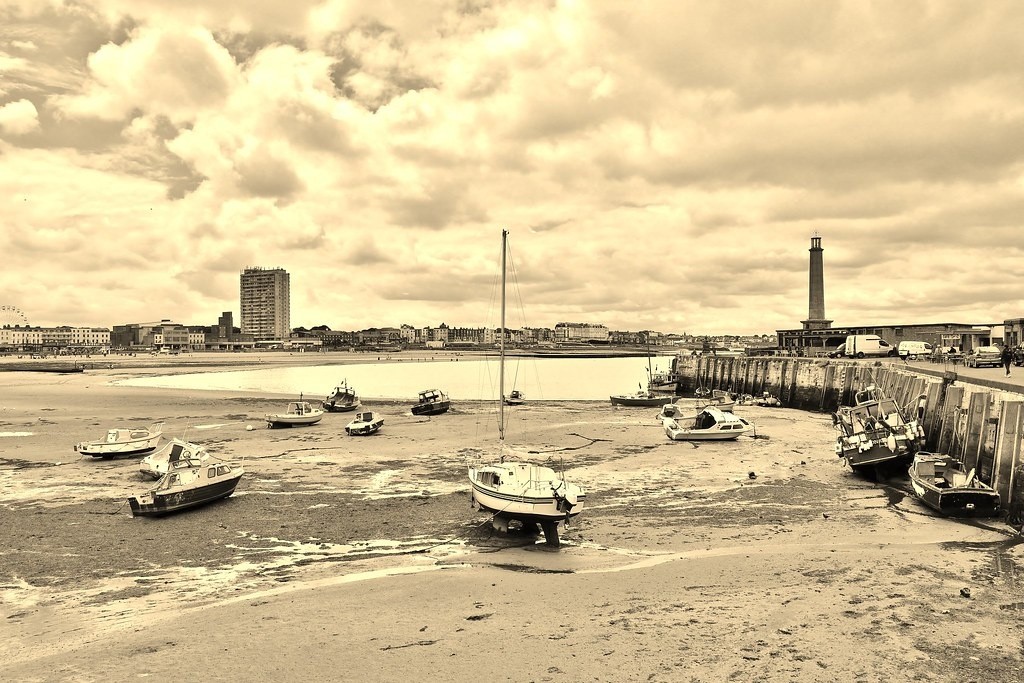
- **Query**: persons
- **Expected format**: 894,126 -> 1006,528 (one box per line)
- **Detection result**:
1001,344 -> 1013,378
934,344 -> 943,364
947,347 -> 957,365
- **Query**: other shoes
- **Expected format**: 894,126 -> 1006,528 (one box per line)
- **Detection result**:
1004,375 -> 1007,378
1008,375 -> 1011,378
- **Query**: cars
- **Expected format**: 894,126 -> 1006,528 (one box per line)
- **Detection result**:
1010,341 -> 1024,366
965,346 -> 1003,367
827,343 -> 846,360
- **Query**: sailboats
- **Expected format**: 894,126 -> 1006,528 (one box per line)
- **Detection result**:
466,228 -> 589,526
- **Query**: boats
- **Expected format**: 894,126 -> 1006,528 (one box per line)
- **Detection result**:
609,390 -> 680,407
830,383 -> 928,473
139,439 -> 210,480
266,400 -> 324,427
694,393 -> 737,415
126,456 -> 246,519
321,379 -> 361,411
662,407 -> 752,441
73,421 -> 163,460
908,450 -> 1002,518
411,389 -> 453,416
345,410 -> 385,437
649,380 -> 679,392
657,403 -> 685,423
696,384 -> 782,408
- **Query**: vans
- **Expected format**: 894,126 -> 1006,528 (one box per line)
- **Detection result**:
898,341 -> 932,360
845,334 -> 895,357
